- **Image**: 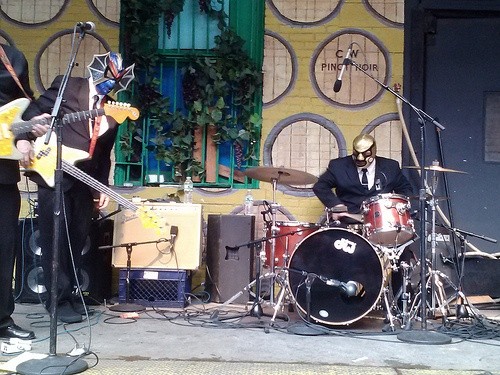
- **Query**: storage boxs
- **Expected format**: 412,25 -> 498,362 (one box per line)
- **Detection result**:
120,269 -> 190,308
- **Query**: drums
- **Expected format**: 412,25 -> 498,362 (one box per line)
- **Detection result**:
361,194 -> 414,245
288,228 -> 384,325
265,220 -> 322,268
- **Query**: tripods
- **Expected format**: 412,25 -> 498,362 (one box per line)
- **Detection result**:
221,178 -> 291,309
351,60 -> 497,329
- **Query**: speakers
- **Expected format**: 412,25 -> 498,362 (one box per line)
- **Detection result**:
110,200 -> 203,269
207,213 -> 256,306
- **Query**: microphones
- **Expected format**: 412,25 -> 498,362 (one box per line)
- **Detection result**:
169,226 -> 178,250
76,21 -> 95,32
333,44 -> 352,92
345,279 -> 359,296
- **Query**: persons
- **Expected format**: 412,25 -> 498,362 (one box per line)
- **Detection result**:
0,43 -> 54,339
15,52 -> 136,323
313,135 -> 421,314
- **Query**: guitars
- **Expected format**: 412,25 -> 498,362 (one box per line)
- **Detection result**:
19,130 -> 167,236
0,98 -> 139,160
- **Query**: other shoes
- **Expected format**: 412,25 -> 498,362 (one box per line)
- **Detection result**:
73,302 -> 89,314
57,310 -> 82,323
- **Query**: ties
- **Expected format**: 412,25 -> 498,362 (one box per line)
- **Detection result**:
362,168 -> 368,191
91,96 -> 100,129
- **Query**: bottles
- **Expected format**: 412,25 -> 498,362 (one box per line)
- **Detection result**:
244,190 -> 253,214
184,177 -> 193,204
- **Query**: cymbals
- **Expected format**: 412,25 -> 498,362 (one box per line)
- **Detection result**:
245,166 -> 318,184
403,165 -> 465,174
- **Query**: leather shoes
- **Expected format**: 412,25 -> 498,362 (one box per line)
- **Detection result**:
0,324 -> 34,340
